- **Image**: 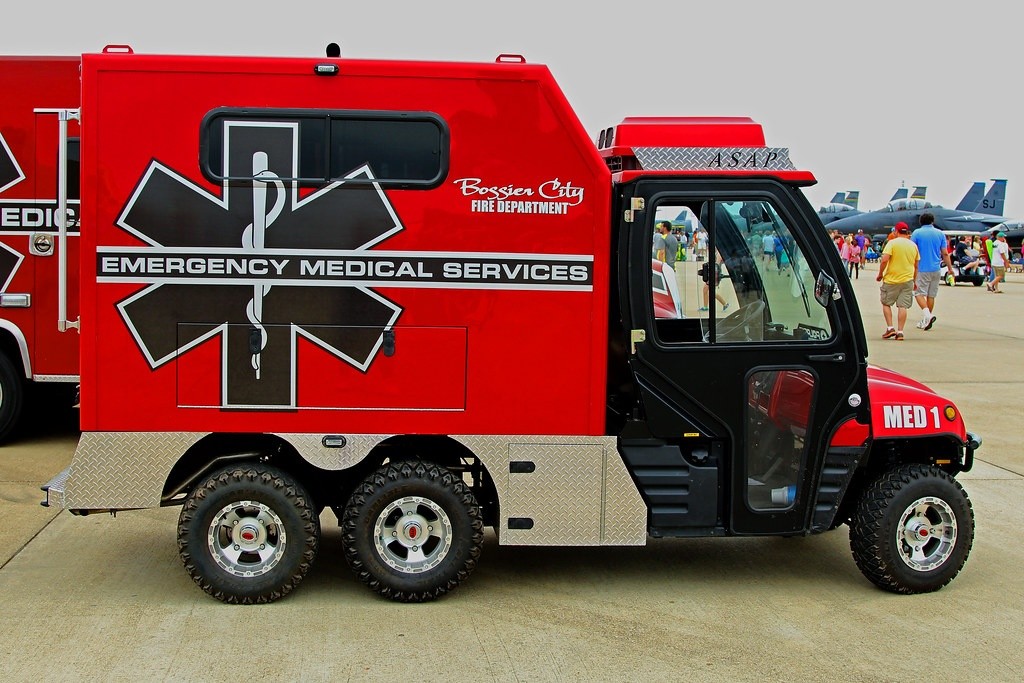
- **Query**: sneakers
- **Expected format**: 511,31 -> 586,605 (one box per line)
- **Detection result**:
923,315 -> 936,330
882,328 -> 897,338
895,332 -> 904,340
917,318 -> 928,329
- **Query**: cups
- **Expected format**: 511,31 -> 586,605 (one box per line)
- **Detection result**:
691,254 -> 696,261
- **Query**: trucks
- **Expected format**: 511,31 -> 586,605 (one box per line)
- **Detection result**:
0,43 -> 686,450
37,50 -> 978,606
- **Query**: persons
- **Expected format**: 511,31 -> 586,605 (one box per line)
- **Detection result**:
911,213 -> 956,331
651,212 -> 1023,342
875,222 -> 920,343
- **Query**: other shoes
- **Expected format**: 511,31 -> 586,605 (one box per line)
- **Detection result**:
988,283 -> 994,292
960,266 -> 965,276
994,289 -> 1002,293
970,271 -> 977,275
697,306 -> 708,311
722,303 -> 730,312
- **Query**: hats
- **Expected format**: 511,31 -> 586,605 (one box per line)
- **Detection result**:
857,229 -> 863,233
997,232 -> 1007,237
764,230 -> 771,235
895,222 -> 908,232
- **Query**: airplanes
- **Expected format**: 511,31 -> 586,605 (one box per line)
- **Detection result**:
655,179 -> 1024,249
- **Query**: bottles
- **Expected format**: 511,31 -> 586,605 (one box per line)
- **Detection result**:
948,274 -> 955,286
